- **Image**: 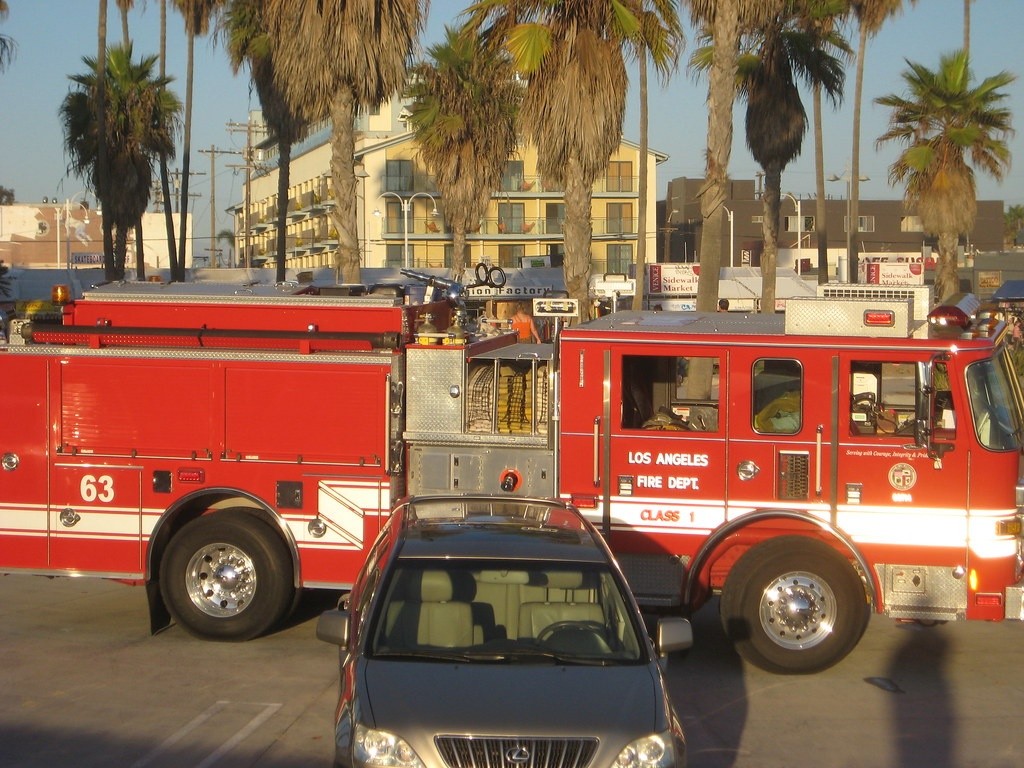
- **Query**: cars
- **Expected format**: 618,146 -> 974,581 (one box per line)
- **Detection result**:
315,493 -> 695,768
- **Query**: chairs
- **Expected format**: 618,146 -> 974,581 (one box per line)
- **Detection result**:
521,222 -> 535,234
495,222 -> 506,230
425,221 -> 445,234
519,180 -> 536,192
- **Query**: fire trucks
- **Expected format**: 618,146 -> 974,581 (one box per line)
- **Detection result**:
1,264 -> 1024,677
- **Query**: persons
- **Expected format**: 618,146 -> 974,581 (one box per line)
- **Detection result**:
654,299 -> 729,312
561,317 -> 568,329
1012,320 -> 1024,350
509,302 -> 542,345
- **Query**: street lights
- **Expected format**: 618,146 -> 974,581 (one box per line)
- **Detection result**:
66,190 -> 102,269
56,202 -> 89,270
669,208 -> 686,264
826,159 -> 873,286
374,192 -> 440,268
